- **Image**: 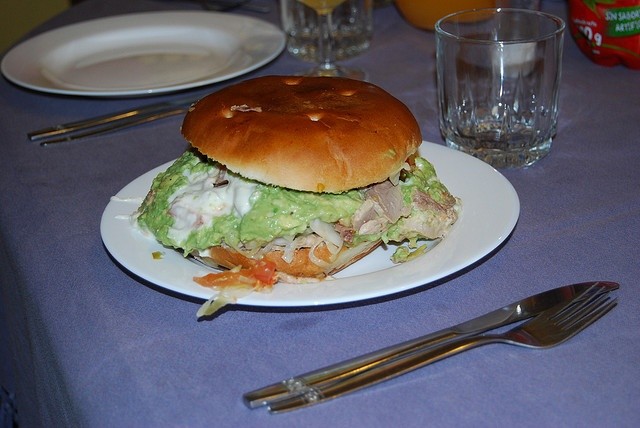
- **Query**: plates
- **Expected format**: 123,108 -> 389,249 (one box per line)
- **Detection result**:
100,142 -> 520,308
1,10 -> 287,96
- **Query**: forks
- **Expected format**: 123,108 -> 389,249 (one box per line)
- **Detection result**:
41,107 -> 189,148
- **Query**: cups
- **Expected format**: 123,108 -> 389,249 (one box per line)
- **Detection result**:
282,1 -> 373,63
433,8 -> 564,170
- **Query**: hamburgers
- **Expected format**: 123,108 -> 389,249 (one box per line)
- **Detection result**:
133,74 -> 461,320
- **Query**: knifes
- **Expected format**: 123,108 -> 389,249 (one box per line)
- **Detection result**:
27,95 -> 200,141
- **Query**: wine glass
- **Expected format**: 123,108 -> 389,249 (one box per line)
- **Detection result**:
300,0 -> 368,82
